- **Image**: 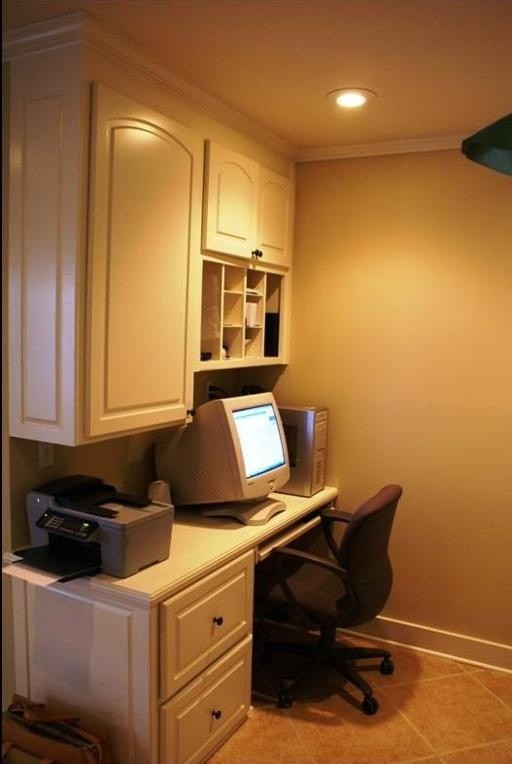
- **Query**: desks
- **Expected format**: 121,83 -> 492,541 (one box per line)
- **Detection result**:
2,486 -> 344,764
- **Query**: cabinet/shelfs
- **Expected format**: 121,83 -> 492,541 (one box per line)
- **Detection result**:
5,47 -> 205,447
195,109 -> 298,372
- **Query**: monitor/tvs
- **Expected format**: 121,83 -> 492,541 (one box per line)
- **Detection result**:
152,390 -> 291,526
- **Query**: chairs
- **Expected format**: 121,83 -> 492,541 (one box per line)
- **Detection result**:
253,485 -> 407,717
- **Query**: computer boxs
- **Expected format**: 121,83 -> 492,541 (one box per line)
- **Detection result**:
274,404 -> 330,498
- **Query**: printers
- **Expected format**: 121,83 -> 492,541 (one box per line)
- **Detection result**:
11,473 -> 175,584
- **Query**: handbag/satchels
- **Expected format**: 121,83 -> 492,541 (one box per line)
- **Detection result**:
0,693 -> 111,764
